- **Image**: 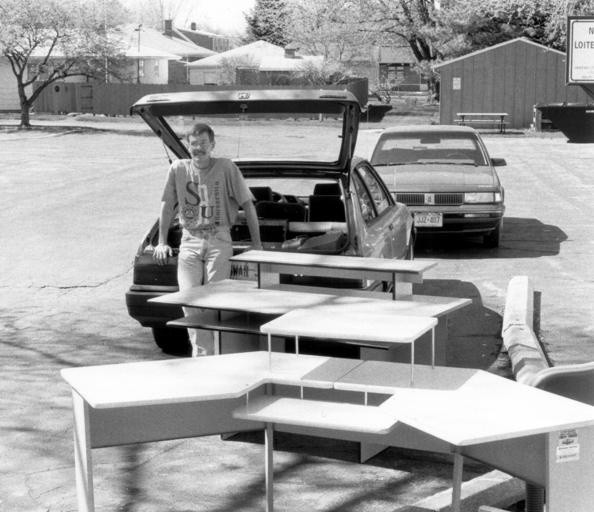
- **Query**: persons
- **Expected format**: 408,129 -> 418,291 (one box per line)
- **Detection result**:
153,122 -> 263,357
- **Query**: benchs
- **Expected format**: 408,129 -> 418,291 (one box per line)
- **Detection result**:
453,112 -> 510,133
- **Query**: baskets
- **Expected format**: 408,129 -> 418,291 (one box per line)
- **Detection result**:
298,231 -> 344,252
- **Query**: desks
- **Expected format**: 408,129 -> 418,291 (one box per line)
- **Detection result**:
146,247 -> 472,462
57,351 -> 594,511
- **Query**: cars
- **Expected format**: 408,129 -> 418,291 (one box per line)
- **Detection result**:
124,89 -> 418,355
369,124 -> 507,250
